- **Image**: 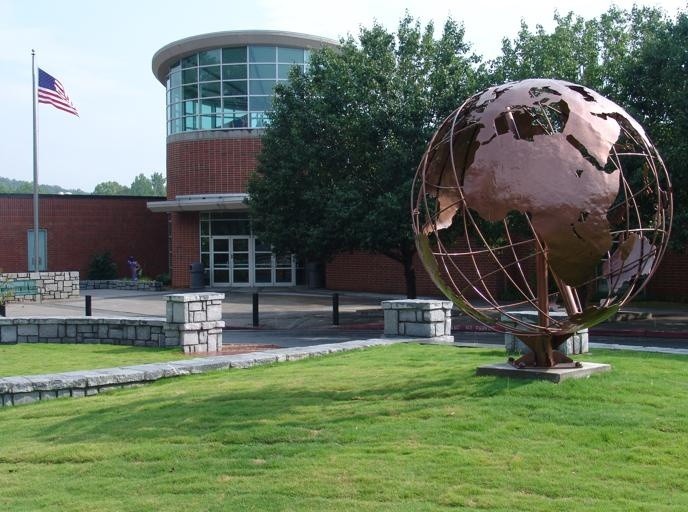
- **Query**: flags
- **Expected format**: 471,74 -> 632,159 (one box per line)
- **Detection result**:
38,68 -> 80,118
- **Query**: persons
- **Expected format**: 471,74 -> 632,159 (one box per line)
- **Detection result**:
127,256 -> 139,281
